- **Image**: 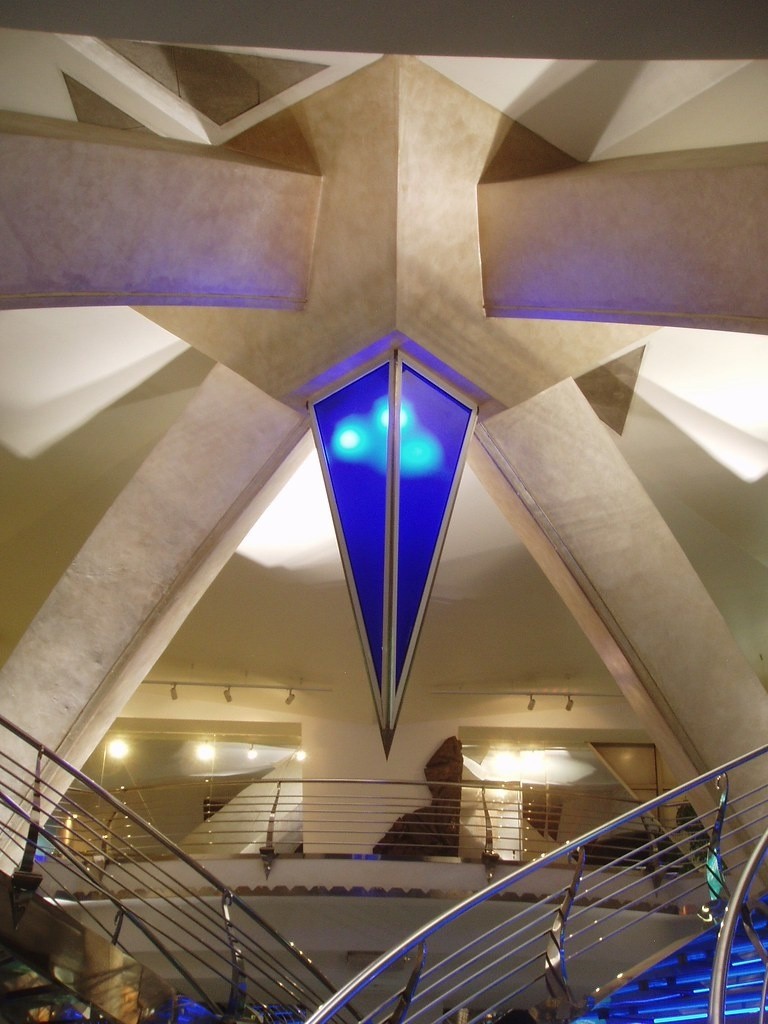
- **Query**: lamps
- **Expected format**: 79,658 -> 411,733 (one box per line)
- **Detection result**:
224,686 -> 233,702
171,683 -> 178,700
565,695 -> 573,711
284,688 -> 294,705
528,694 -> 535,710
